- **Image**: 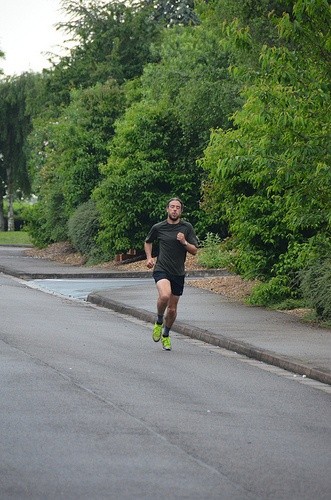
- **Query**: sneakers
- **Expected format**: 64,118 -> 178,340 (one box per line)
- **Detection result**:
161,335 -> 172,351
153,321 -> 162,342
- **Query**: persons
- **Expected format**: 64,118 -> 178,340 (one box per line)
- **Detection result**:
143,198 -> 198,351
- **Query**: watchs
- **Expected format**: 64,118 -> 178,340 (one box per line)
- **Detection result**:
182,240 -> 187,246
